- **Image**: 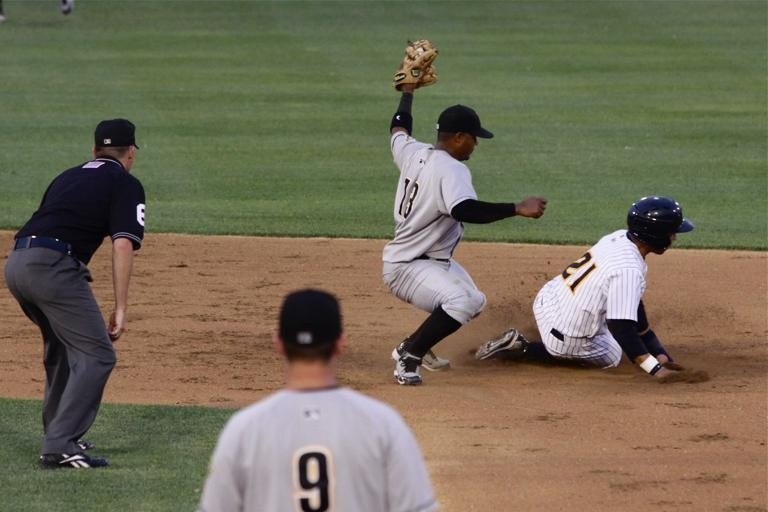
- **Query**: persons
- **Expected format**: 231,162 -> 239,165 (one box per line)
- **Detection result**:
373,37 -> 552,385
192,283 -> 441,510
2,117 -> 148,470
471,193 -> 694,379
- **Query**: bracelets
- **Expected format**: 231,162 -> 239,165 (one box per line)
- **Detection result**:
649,364 -> 663,376
639,355 -> 660,373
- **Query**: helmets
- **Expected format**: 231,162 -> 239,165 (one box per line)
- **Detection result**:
627,195 -> 694,255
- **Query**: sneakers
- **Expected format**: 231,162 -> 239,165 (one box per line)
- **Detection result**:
40,439 -> 107,469
475,328 -> 528,360
392,338 -> 449,385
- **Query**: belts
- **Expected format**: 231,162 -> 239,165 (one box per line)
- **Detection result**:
15,238 -> 66,253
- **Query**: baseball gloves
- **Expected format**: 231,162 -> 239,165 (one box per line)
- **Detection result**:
393,39 -> 438,90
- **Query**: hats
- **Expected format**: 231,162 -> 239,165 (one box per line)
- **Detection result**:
279,291 -> 342,346
436,105 -> 494,138
95,119 -> 138,148
